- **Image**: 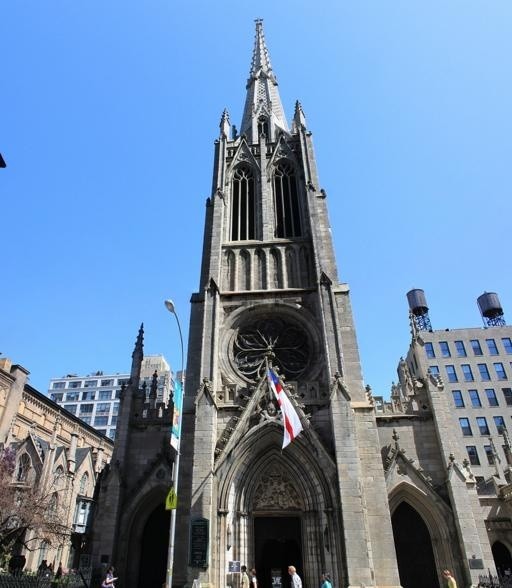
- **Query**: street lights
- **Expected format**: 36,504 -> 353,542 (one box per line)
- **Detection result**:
165,297 -> 186,588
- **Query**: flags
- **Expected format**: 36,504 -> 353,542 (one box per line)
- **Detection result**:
266,367 -> 304,449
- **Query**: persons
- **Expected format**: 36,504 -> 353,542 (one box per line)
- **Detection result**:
249,569 -> 258,588
37,560 -> 62,581
105,566 -> 118,588
442,569 -> 457,588
319,573 -> 333,588
288,565 -> 302,588
240,565 -> 249,588
